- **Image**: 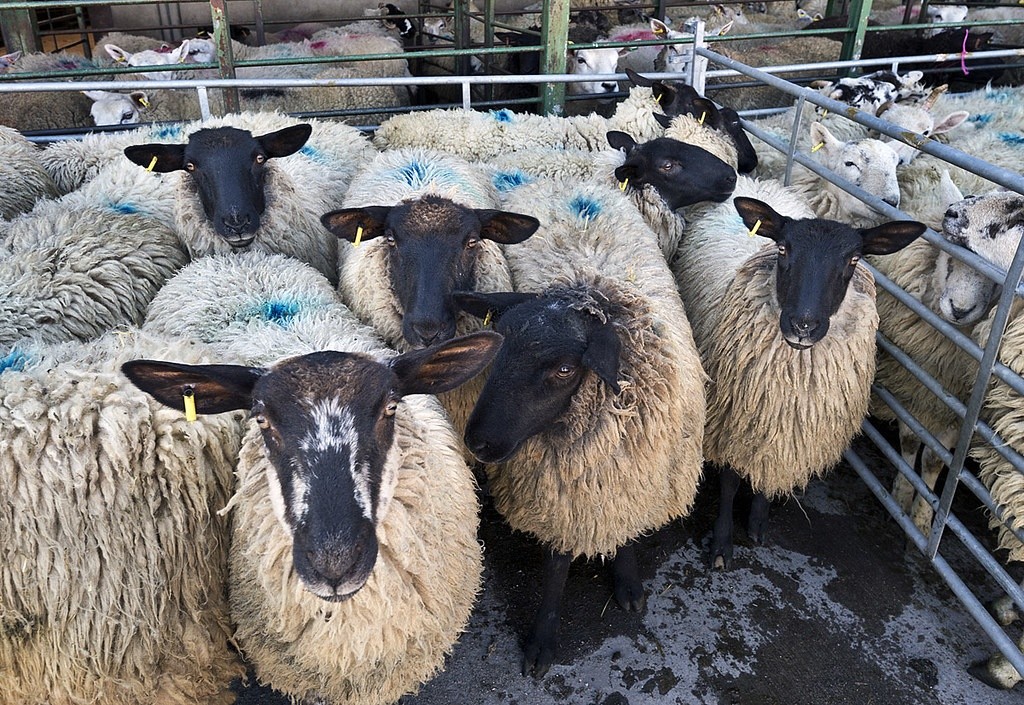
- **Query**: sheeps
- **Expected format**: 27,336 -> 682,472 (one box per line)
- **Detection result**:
1,1 -> 1024,705
